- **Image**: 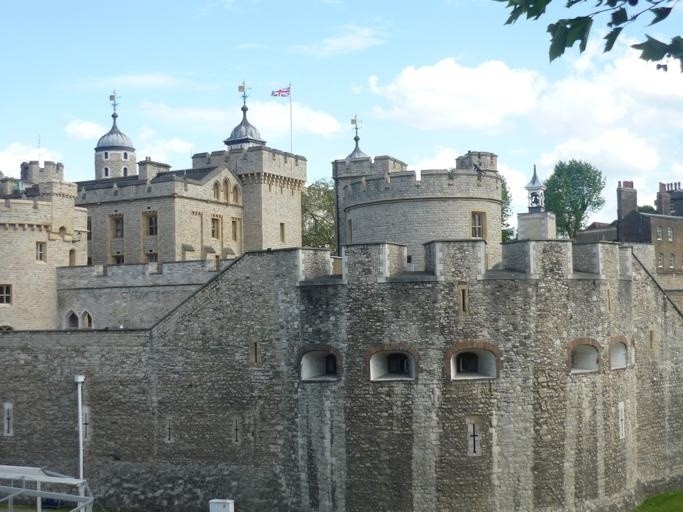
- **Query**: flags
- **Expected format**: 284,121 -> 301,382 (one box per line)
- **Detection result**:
271,86 -> 290,96
